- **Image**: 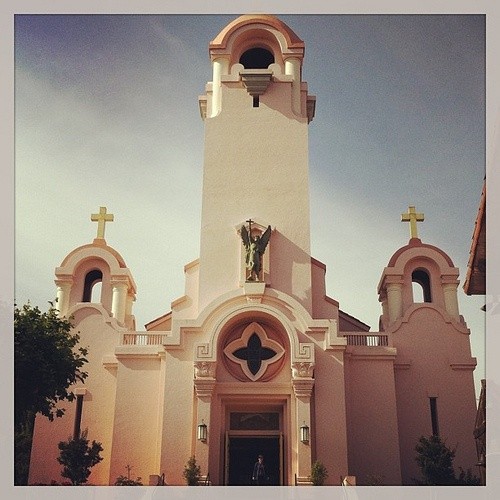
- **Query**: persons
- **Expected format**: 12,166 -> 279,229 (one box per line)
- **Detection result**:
252,454 -> 270,485
241,225 -> 271,281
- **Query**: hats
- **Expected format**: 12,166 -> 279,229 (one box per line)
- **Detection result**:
258,454 -> 263,458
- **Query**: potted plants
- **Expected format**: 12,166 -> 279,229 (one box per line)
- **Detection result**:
414,431 -> 460,486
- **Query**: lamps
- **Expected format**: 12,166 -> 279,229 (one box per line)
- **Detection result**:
197,418 -> 207,442
300,421 -> 310,444
